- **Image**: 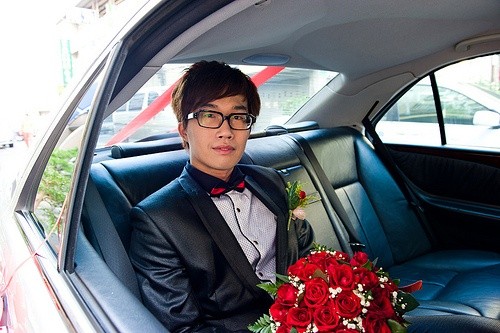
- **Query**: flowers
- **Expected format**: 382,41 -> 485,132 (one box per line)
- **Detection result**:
247,241 -> 422,333
285,179 -> 320,232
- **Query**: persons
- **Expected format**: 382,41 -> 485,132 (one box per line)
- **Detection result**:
128,59 -> 316,333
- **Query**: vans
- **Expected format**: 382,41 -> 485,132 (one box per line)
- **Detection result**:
111,91 -> 179,133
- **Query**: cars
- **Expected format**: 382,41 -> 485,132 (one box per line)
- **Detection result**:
376,79 -> 497,149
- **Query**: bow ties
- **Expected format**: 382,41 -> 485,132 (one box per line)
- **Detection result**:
210,176 -> 246,197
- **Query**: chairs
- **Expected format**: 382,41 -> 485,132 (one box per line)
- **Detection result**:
84,126 -> 500,333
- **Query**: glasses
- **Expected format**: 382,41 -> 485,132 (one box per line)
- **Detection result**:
186,109 -> 256,130
